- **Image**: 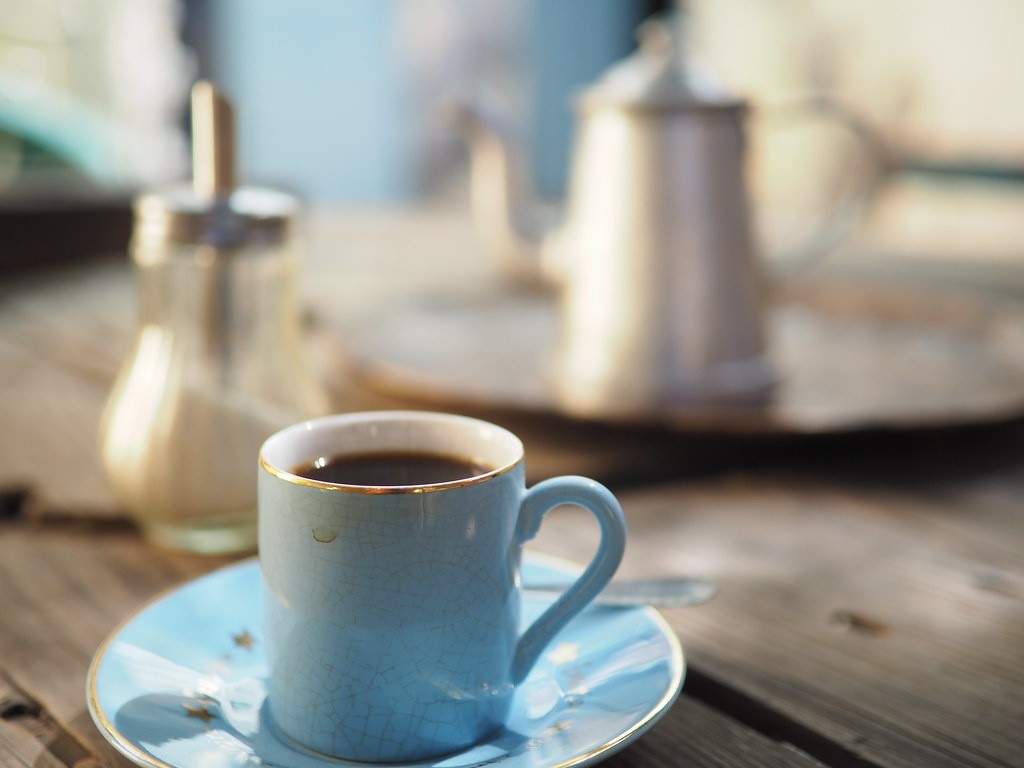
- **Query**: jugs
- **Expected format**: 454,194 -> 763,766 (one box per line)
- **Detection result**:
449,16 -> 775,418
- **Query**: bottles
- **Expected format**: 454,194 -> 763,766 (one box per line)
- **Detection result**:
96,186 -> 328,557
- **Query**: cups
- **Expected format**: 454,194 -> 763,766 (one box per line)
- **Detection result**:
259,411 -> 629,765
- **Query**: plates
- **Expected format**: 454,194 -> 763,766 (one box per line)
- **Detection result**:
83,548 -> 685,768
299,257 -> 1020,448
